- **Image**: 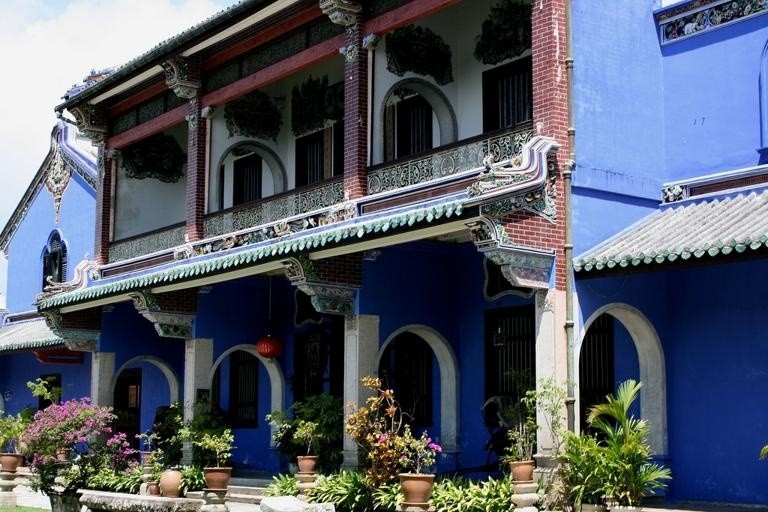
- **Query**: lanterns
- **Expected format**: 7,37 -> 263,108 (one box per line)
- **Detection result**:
256,332 -> 283,363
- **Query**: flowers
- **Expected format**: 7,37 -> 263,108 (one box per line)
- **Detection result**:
378,423 -> 443,475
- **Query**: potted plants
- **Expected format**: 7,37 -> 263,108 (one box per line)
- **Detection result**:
146,461 -> 162,496
295,419 -> 323,474
566,374 -> 676,512
135,432 -> 161,466
192,429 -> 233,488
499,415 -> 543,481
553,426 -> 635,511
0,412 -> 29,472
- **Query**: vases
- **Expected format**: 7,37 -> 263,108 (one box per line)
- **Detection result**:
397,472 -> 435,504
